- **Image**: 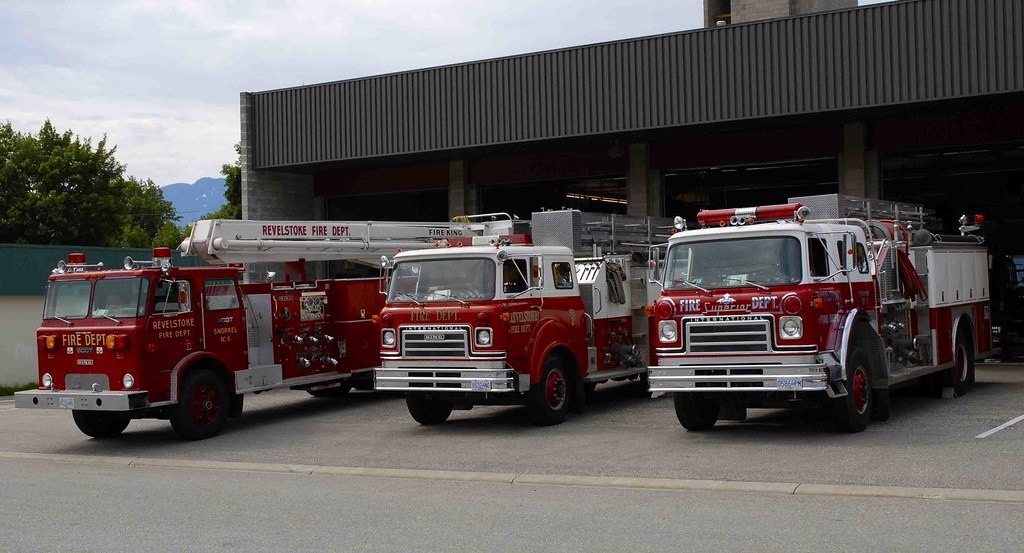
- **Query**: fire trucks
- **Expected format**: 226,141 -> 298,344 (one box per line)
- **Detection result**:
644,192 -> 1004,432
372,207 -> 666,426
13,219 -> 488,441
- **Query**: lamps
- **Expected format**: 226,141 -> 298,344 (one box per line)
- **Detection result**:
607,135 -> 625,159
